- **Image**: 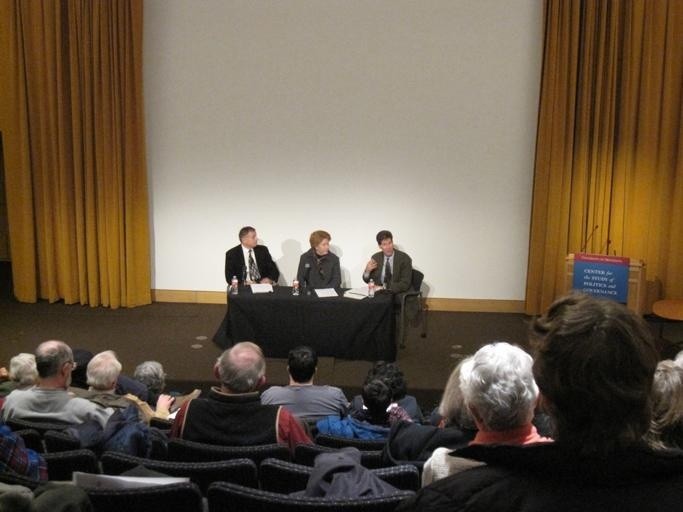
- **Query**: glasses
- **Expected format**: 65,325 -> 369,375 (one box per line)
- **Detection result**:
65,360 -> 78,370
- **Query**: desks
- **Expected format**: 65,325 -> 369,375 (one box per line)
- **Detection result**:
211,285 -> 397,363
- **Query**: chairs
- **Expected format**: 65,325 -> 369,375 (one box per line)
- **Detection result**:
166,436 -> 294,479
207,481 -> 417,511
291,443 -> 382,470
158,429 -> 171,441
9,429 -> 45,453
101,450 -> 260,499
304,419 -> 318,440
394,269 -> 426,348
148,416 -> 174,431
0,470 -> 204,512
43,430 -> 85,454
313,432 -> 388,451
258,456 -> 419,495
4,418 -> 80,441
35,448 -> 100,481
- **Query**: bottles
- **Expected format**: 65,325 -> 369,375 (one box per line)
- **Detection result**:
368,278 -> 375,298
293,277 -> 299,295
232,275 -> 238,295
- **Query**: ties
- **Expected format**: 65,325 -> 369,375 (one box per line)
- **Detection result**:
384,259 -> 392,286
248,249 -> 261,281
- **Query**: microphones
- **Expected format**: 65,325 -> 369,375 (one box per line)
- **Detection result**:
241,265 -> 247,281
579,225 -> 598,253
303,262 -> 309,283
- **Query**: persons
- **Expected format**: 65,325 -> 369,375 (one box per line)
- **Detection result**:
225,226 -> 278,286
421,342 -> 554,489
400,296 -> 682,511
0,422 -> 49,482
1,484 -> 93,507
86,349 -> 147,424
645,360 -> 682,448
297,231 -> 341,289
366,363 -> 426,416
317,378 -> 414,442
2,353 -> 37,396
425,360 -> 479,453
1,339 -> 114,430
260,347 -> 349,418
172,342 -> 312,446
362,230 -> 420,321
71,348 -> 94,390
132,360 -> 180,398
